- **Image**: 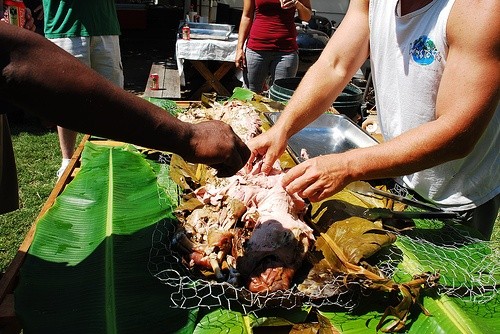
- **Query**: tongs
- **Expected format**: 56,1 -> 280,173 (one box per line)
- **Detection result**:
346,176 -> 446,213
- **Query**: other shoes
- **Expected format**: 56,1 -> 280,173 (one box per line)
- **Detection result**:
57,166 -> 68,182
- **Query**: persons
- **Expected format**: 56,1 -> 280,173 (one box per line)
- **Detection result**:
243,0 -> 500,241
0,0 -> 46,37
0,21 -> 251,214
235,0 -> 312,95
42,0 -> 125,183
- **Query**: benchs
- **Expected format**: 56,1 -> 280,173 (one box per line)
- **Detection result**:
144,62 -> 181,99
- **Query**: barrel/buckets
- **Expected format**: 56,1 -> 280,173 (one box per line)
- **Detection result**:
269,76 -> 364,119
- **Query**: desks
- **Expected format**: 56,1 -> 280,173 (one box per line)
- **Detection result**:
175,32 -> 247,98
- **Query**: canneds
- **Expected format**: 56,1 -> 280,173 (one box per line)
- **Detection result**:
182,27 -> 190,40
1,0 -> 27,29
150,74 -> 159,90
